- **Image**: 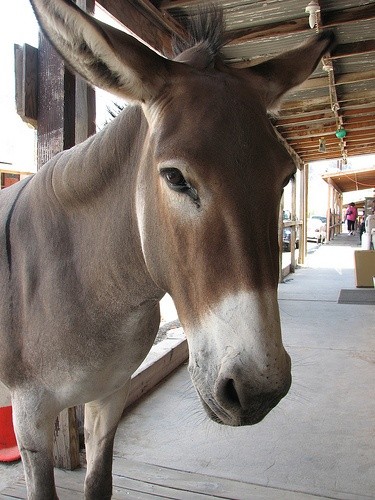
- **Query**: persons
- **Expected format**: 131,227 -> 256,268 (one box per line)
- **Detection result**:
343,202 -> 357,236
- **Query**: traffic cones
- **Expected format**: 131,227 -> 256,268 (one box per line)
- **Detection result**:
0,380 -> 22,464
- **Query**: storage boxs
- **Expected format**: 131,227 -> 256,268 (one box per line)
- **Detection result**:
355,250 -> 375,288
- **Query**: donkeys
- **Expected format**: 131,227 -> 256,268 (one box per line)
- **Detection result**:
1,1 -> 333,500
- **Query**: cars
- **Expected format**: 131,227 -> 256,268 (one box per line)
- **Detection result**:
307,215 -> 327,243
282,209 -> 300,252
